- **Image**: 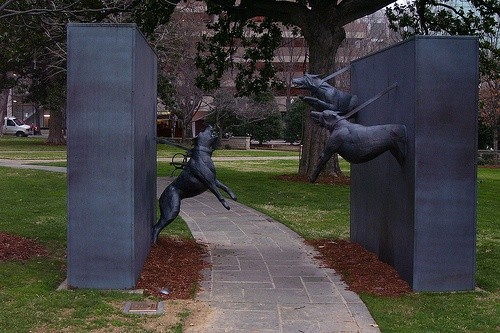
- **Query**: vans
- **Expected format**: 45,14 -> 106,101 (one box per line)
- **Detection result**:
3,117 -> 32,137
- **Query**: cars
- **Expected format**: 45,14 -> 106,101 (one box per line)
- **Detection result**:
19,119 -> 41,136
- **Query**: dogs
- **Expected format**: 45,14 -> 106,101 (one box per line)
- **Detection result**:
152,124 -> 237,244
292,73 -> 359,119
311,109 -> 406,184
